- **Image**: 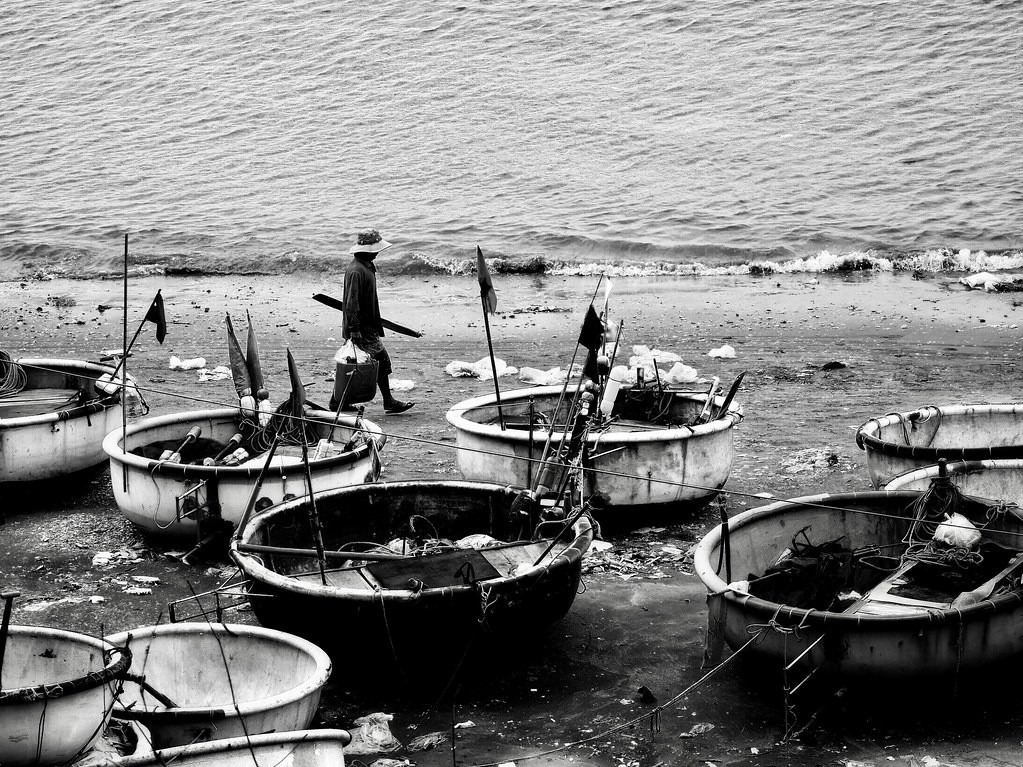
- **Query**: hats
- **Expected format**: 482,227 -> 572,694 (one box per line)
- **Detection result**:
350,228 -> 394,254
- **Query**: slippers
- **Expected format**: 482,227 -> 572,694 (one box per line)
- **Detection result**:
329,402 -> 358,413
385,401 -> 415,414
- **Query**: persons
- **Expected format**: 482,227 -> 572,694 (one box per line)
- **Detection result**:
328,227 -> 415,414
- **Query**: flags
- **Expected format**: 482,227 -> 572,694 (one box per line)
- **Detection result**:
145,294 -> 167,346
577,276 -> 613,384
700,595 -> 727,668
225,308 -> 265,396
476,244 -> 497,314
286,347 -> 307,405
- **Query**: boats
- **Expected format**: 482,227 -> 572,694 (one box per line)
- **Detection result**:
3,623 -> 133,766
101,618 -> 334,742
858,402 -> 1023,515
694,488 -> 1023,672
0,354 -> 138,484
87,725 -> 350,767
230,475 -> 594,652
446,384 -> 744,516
515,488 -> 693,652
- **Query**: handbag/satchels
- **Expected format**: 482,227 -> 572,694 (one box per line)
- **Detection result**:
335,359 -> 378,402
